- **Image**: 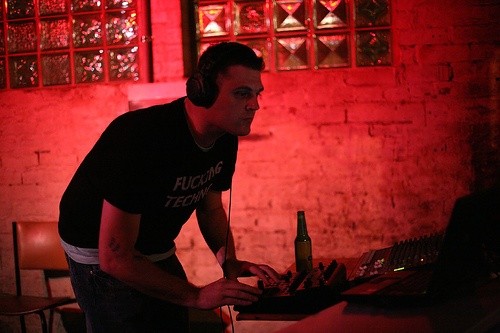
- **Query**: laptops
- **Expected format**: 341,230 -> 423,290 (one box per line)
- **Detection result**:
341,188 -> 500,306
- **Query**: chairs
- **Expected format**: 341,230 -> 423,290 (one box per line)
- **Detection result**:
0,220 -> 77,333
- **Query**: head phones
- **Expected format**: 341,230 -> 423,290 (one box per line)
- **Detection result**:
186,42 -> 228,106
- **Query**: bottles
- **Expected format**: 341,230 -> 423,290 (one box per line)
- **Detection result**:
294,210 -> 313,276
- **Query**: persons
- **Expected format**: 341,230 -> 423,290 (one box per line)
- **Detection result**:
57,42 -> 281,333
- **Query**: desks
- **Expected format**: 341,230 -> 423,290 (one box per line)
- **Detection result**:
236,256 -> 355,321
272,272 -> 500,333
